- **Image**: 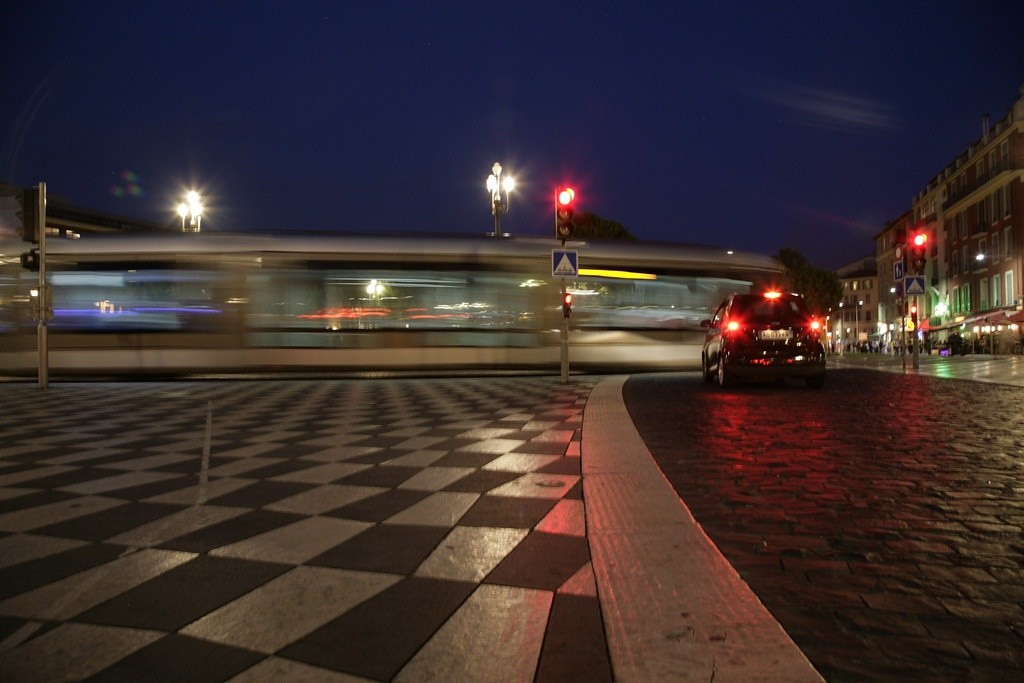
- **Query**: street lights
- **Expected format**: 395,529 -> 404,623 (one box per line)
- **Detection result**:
855,299 -> 866,350
977,249 -> 987,354
837,299 -> 851,355
173,176 -> 207,241
890,286 -> 901,355
486,159 -> 519,249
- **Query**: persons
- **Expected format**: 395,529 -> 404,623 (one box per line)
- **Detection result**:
847,332 -> 999,357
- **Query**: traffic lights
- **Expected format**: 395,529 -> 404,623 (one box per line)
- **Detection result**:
555,184 -> 577,241
562,291 -> 573,319
911,305 -> 918,323
909,225 -> 929,272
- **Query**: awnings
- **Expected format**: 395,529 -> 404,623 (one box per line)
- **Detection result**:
869,331 -> 893,337
927,310 -> 1024,333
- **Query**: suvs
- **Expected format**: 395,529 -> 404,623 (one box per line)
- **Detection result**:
698,293 -> 827,393
